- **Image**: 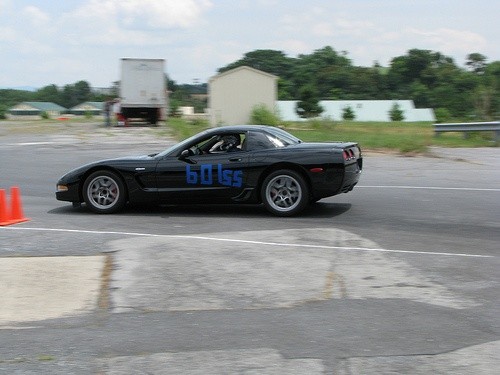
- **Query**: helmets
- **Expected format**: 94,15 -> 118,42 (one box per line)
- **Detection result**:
217,134 -> 241,150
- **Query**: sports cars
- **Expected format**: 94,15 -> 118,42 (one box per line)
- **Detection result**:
55,122 -> 364,218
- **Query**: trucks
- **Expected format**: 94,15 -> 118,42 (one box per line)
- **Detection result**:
118,56 -> 168,126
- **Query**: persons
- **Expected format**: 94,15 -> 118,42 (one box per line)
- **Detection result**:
196,133 -> 242,156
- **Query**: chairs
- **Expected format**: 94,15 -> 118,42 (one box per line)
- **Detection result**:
220,132 -> 242,152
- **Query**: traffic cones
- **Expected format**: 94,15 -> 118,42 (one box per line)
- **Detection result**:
9,186 -> 29,224
0,188 -> 16,227
